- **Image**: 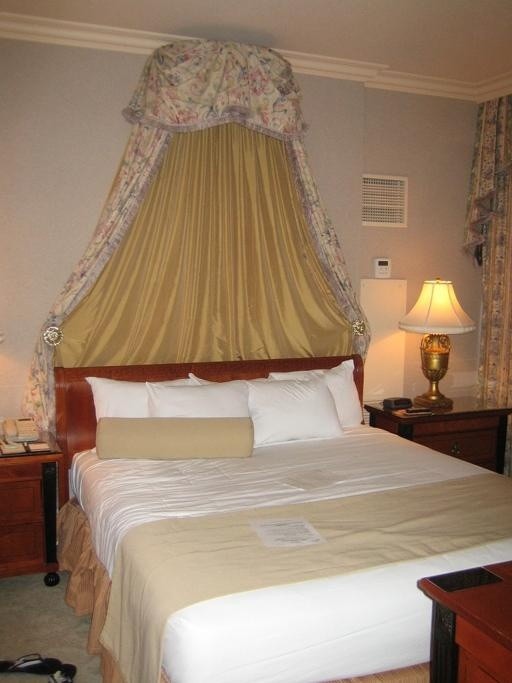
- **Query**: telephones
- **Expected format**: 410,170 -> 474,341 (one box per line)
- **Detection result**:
3,417 -> 39,442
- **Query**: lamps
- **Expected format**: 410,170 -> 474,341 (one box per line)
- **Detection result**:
397,279 -> 477,407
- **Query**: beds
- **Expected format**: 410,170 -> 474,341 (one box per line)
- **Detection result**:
32,354 -> 510,683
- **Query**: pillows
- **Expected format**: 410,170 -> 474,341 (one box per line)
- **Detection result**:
86,374 -> 194,417
146,377 -> 247,424
272,358 -> 365,429
97,416 -> 255,459
241,379 -> 345,447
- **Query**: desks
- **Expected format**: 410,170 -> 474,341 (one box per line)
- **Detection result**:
414,557 -> 512,683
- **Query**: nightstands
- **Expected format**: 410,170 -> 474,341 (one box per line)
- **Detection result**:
365,393 -> 512,474
0,430 -> 67,586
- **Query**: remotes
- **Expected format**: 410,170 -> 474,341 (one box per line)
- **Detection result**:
406,409 -> 432,415
